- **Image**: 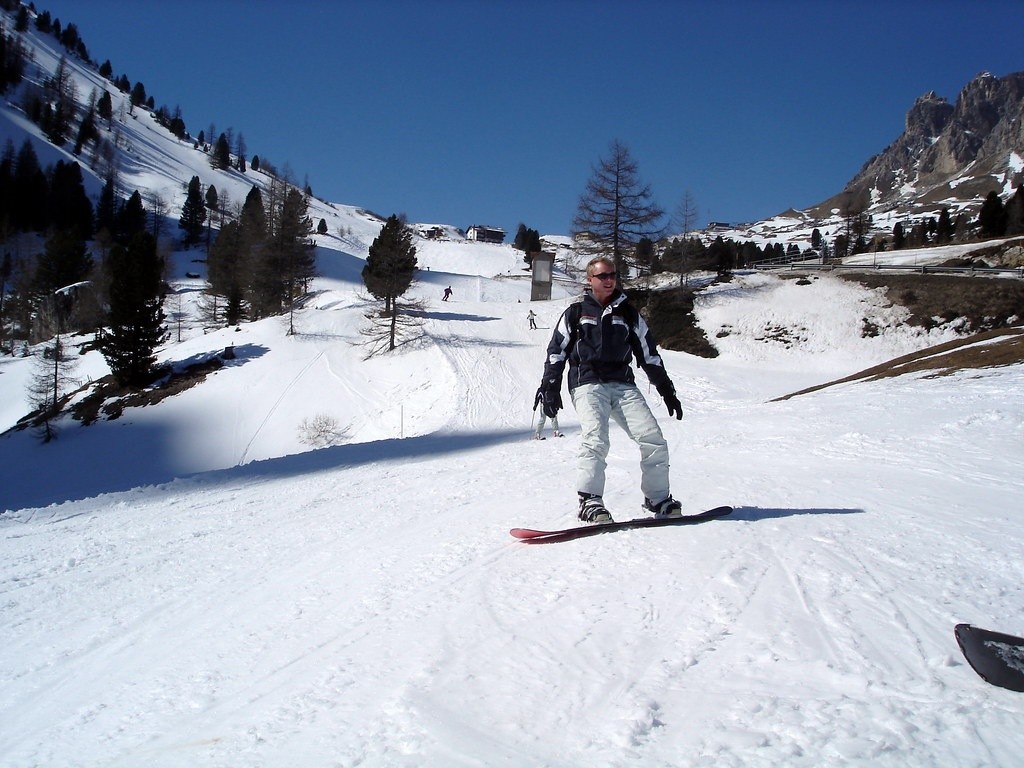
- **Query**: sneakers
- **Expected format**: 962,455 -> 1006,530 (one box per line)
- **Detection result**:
642,496 -> 681,517
579,495 -> 611,522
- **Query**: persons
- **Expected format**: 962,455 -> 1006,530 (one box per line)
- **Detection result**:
527,310 -> 536,329
533,379 -> 563,439
543,257 -> 683,524
442,286 -> 453,300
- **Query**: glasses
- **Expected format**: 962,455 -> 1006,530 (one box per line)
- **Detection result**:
592,272 -> 618,279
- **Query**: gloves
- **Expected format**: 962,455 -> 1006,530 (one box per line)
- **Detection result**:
533,406 -> 536,411
657,380 -> 684,420
535,384 -> 563,418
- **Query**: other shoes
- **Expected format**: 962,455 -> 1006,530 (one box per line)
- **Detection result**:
554,432 -> 563,437
537,435 -> 546,440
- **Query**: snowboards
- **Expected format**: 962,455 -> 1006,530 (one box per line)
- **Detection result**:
954,624 -> 1024,693
509,506 -> 732,541
